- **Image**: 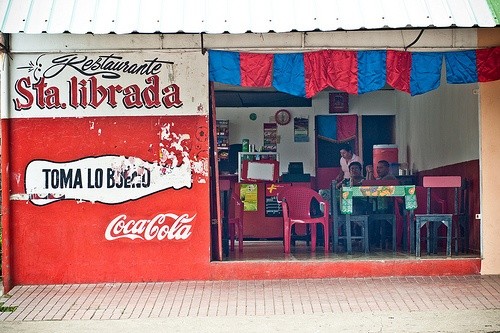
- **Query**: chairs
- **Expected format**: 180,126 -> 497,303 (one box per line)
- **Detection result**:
222,190 -> 245,256
278,176 -> 460,257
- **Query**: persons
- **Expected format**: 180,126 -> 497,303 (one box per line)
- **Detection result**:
336,162 -> 366,249
336,144 -> 364,182
353,160 -> 403,249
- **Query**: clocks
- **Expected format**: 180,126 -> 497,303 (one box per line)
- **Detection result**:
275,110 -> 290,125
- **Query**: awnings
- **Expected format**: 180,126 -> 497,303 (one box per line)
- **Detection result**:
0,0 -> 500,36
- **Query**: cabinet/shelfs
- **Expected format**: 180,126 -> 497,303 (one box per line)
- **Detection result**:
215,119 -> 230,156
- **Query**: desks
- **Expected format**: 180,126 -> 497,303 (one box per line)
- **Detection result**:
340,185 -> 416,254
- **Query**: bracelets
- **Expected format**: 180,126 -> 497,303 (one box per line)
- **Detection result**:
369,171 -> 373,173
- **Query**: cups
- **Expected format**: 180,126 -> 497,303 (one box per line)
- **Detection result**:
404,168 -> 409,175
399,168 -> 404,176
242,139 -> 255,153
410,169 -> 413,175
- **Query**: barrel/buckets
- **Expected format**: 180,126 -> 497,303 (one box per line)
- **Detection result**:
373,144 -> 398,178
